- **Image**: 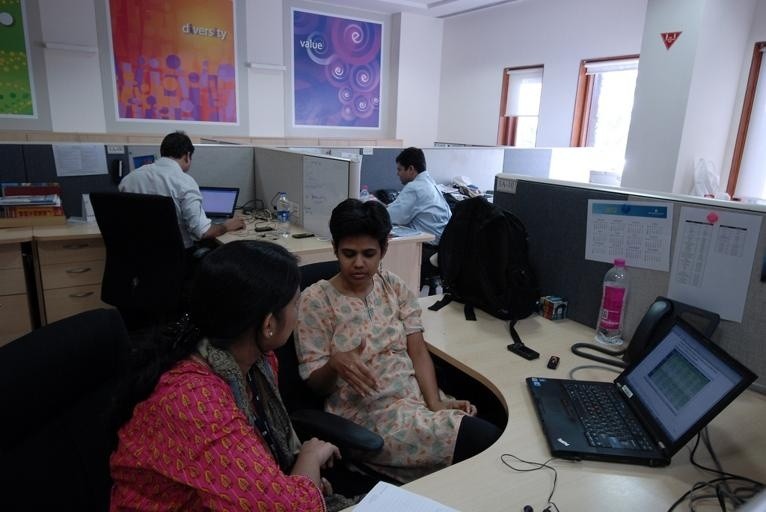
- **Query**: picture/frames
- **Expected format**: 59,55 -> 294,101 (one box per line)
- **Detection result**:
94,0 -> 250,138
281,0 -> 384,140
1,1 -> 53,134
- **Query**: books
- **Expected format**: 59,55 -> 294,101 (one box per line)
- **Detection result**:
0,183 -> 70,231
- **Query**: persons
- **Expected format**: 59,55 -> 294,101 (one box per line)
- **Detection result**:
100,236 -> 343,511
113,131 -> 248,258
369,147 -> 453,292
289,196 -> 502,483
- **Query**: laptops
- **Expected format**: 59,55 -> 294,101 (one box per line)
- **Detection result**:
198,186 -> 240,225
525,316 -> 759,469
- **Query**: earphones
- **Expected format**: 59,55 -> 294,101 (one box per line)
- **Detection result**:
262,233 -> 266,237
542,506 -> 552,512
272,237 -> 278,240
523,505 -> 533,512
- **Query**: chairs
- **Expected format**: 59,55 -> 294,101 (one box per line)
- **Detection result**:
92,189 -> 182,320
272,259 -> 412,495
0,303 -> 146,510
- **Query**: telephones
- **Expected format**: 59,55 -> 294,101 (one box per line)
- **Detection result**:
376,188 -> 399,205
622,296 -> 720,370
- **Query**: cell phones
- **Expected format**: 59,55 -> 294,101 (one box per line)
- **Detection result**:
292,232 -> 314,238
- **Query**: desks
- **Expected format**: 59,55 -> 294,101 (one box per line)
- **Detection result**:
30,203 -> 336,331
379,188 -> 492,294
324,293 -> 765,511
0,219 -> 34,344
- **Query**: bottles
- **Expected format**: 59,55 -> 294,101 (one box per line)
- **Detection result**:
596,258 -> 631,343
277,191 -> 291,239
360,185 -> 369,199
